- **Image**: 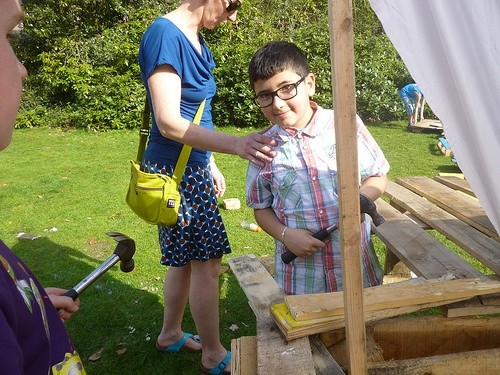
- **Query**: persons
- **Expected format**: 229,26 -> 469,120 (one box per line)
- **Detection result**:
400,83 -> 426,128
247,40 -> 391,296
138,0 -> 278,375
0,0 -> 89,375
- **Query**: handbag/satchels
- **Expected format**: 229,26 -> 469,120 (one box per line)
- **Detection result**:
125,160 -> 181,226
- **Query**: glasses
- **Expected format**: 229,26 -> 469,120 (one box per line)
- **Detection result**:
252,75 -> 307,109
226,0 -> 242,12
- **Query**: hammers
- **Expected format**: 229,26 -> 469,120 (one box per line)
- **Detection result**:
56,230 -> 136,315
280,192 -> 386,265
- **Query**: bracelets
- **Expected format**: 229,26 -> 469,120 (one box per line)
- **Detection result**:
281,227 -> 290,244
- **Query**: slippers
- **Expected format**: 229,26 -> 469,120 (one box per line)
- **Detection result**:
156,331 -> 202,354
199,350 -> 232,375
